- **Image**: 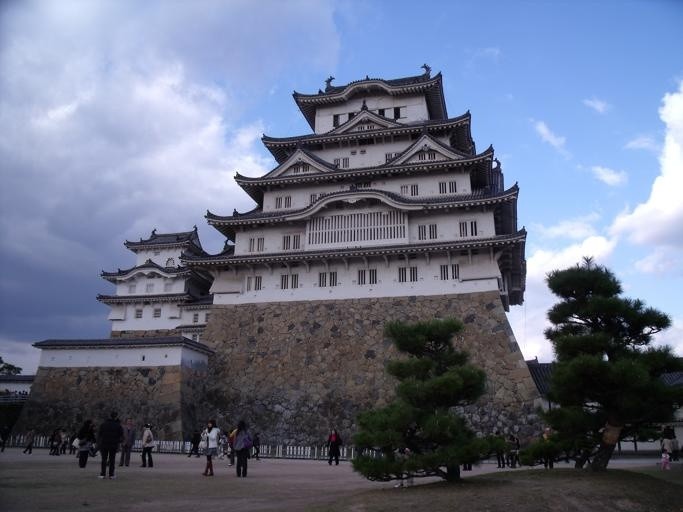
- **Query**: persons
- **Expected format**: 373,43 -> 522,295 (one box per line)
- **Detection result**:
21,428 -> 36,455
660,449 -> 670,470
671,436 -> 680,461
94,411 -> 124,479
0,424 -> 9,452
186,427 -> 202,458
541,427 -> 553,469
220,429 -> 261,465
463,461 -> 471,470
325,428 -> 342,466
492,428 -> 520,468
200,419 -> 222,476
390,445 -> 416,489
233,420 -> 253,477
44,417 -> 153,468
661,437 -> 672,460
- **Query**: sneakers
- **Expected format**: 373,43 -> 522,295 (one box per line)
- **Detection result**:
208,474 -> 214,476
202,473 -> 207,476
109,477 -> 116,480
97,475 -> 104,479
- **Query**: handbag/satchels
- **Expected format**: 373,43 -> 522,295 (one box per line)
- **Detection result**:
78,440 -> 87,447
72,437 -> 79,448
244,437 -> 254,449
199,440 -> 208,450
147,436 -> 152,444
